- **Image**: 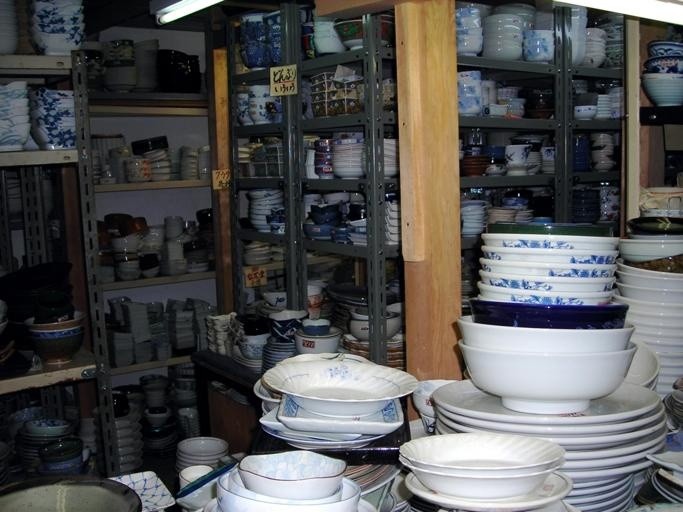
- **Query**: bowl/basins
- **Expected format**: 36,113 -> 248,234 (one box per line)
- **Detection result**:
0,289 -> 84,367
234,71 -> 394,125
261,278 -> 403,345
90,136 -> 210,182
97,204 -> 219,284
237,134 -> 400,181
4,173 -> 58,216
215,451 -> 361,511
239,13 -> 393,67
245,186 -> 400,246
415,379 -> 454,433
461,185 -> 622,240
454,233 -> 639,415
400,433 -> 569,501
458,41 -> 682,121
105,295 -> 344,374
0,4 -> 83,151
84,37 -> 202,93
459,132 -> 618,178
459,2 -> 626,68
175,437 -> 229,477
1,363 -> 206,479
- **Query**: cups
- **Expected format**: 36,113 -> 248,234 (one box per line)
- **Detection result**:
178,465 -> 215,490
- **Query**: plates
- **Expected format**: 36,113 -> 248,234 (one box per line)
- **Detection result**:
251,352 -> 422,446
638,393 -> 683,511
242,241 -> 316,266
433,379 -> 666,512
404,470 -> 573,509
614,211 -> 682,401
2,475 -> 143,511
